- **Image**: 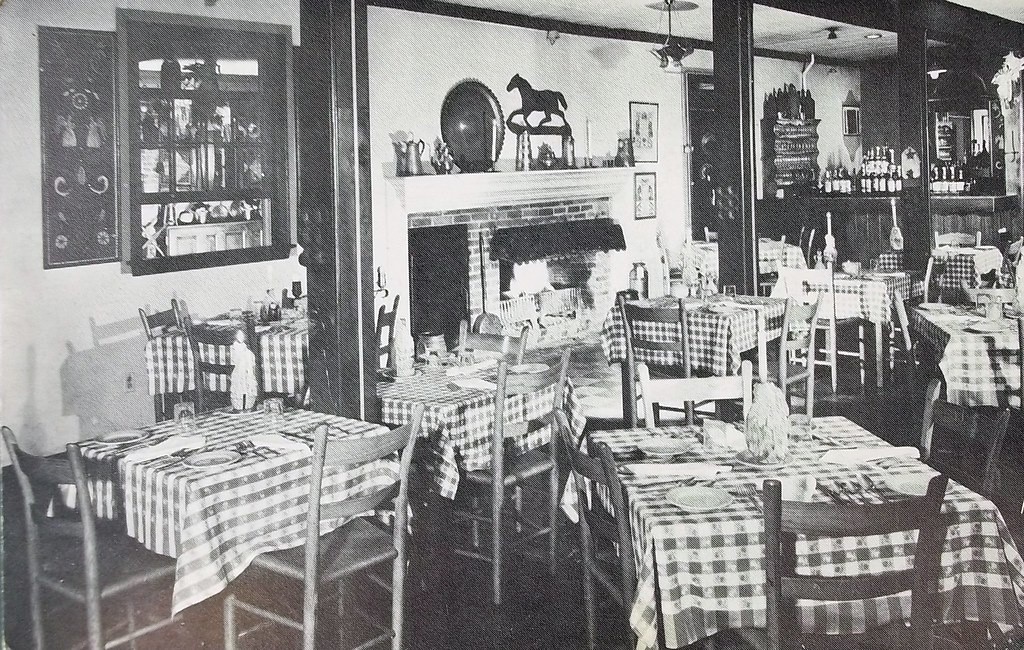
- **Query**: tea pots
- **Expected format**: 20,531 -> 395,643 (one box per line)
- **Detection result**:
403,138 -> 425,175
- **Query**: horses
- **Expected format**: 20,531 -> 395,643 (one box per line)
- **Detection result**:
506,74 -> 569,127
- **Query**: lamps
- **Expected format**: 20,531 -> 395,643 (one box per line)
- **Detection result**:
643,0 -> 700,67
826,26 -> 839,39
928,60 -> 946,81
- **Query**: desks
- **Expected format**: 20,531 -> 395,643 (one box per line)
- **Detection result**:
681,239 -> 807,296
146,296 -> 389,424
597,296 -> 799,421
49,405 -> 405,649
929,247 -> 1004,303
580,409 -> 1024,650
372,360 -> 594,546
907,304 -> 1024,481
772,267 -> 922,388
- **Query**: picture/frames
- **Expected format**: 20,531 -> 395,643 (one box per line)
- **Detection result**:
634,172 -> 657,220
628,101 -> 659,162
38,25 -> 121,269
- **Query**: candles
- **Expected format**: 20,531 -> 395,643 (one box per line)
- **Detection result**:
491,119 -> 496,160
586,117 -> 591,159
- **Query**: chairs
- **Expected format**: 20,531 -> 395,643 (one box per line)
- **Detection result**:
1,235 -> 1023,649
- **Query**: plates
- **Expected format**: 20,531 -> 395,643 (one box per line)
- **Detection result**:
919,303 -> 952,310
183,451 -> 241,471
510,363 -> 550,373
885,473 -> 955,496
666,486 -> 732,511
736,450 -> 793,468
974,246 -> 997,248
382,369 -> 422,380
220,404 -> 264,417
970,325 -> 1006,333
94,429 -> 148,445
709,306 -> 739,312
637,438 -> 691,456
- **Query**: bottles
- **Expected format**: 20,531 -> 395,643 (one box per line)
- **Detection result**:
929,164 -> 973,196
824,146 -> 902,194
615,137 -> 633,167
413,331 -> 448,361
260,290 -> 281,321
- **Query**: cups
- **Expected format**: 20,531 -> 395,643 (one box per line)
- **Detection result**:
788,414 -> 814,454
703,420 -> 726,453
723,286 -> 736,308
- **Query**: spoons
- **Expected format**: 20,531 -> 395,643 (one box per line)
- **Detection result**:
827,473 -> 889,507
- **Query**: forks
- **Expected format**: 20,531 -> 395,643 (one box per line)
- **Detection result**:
737,482 -> 765,514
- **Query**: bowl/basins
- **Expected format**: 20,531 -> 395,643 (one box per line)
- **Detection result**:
842,262 -> 861,274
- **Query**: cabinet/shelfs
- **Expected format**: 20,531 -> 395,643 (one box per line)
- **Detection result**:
136,85 -> 265,202
762,119 -> 823,196
934,116 -> 954,160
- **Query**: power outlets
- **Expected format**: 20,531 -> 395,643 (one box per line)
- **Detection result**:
120,372 -> 136,393
